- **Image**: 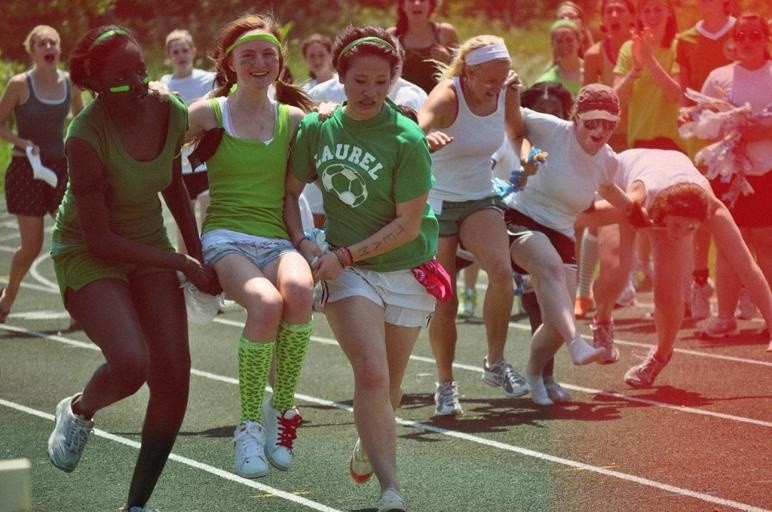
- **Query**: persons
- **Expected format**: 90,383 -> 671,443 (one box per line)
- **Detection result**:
47,25 -> 223,512
145,14 -> 340,479
160,28 -> 235,313
0,25 -> 84,331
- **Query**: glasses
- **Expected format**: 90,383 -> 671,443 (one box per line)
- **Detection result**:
580,119 -> 618,131
734,30 -> 766,46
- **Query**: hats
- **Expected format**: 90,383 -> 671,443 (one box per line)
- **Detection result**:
574,82 -> 622,124
26,144 -> 60,192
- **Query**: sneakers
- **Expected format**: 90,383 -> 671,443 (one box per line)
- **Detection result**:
456,290 -> 481,319
374,486 -> 410,512
613,277 -> 640,309
429,379 -> 470,422
542,377 -> 573,407
229,400 -> 304,483
345,435 -> 377,486
634,254 -> 658,280
683,278 -> 765,344
0,285 -> 14,329
482,352 -> 532,401
44,395 -> 98,478
586,312 -> 625,365
567,286 -> 596,319
623,346 -> 677,391
